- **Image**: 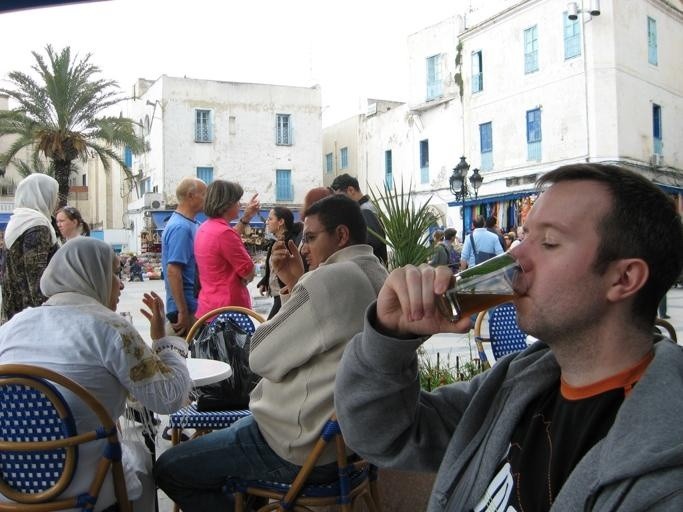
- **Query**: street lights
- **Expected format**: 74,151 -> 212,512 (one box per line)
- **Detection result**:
450,155 -> 484,243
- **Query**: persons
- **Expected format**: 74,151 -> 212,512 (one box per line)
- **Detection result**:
1,237 -> 196,511
152,195 -> 390,512
333,164 -> 683,511
116,251 -> 143,281
1,172 -> 91,322
161,174 -> 388,334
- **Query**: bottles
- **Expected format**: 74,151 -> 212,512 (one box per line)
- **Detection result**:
120,311 -> 133,324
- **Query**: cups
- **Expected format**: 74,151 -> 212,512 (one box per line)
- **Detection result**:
434,252 -> 530,324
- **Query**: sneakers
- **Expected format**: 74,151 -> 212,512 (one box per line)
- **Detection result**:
162,426 -> 190,441
125,408 -> 161,425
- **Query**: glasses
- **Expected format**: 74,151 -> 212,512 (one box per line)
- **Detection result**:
63,206 -> 79,219
115,266 -> 122,279
300,230 -> 326,244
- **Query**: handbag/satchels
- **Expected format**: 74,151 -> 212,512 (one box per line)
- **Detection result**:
191,317 -> 253,412
475,251 -> 497,265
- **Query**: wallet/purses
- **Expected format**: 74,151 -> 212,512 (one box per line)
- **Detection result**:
166,310 -> 187,337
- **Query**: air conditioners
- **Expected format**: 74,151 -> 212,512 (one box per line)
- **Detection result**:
150,197 -> 167,211
